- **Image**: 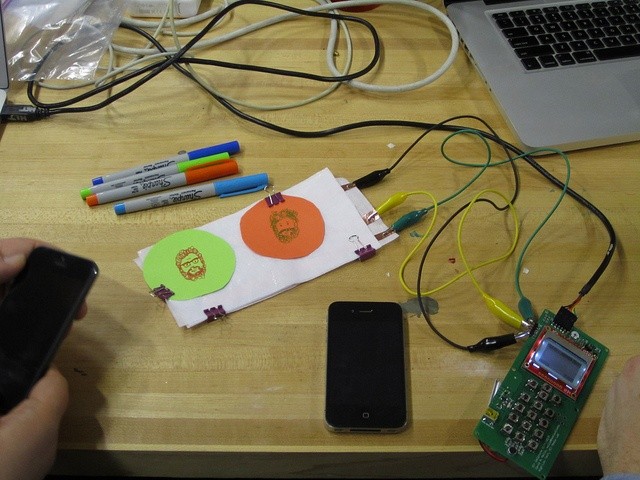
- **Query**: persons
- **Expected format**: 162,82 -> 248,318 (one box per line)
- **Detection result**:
596,354 -> 640,478
0,237 -> 90,480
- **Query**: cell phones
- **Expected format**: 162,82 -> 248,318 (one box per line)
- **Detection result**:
1,246 -> 98,420
326,300 -> 409,435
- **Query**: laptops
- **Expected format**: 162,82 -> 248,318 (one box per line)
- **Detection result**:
440,0 -> 634,157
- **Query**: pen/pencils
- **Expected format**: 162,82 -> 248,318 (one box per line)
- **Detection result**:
83,161 -> 240,208
80,151 -> 231,200
114,174 -> 269,216
92,140 -> 238,185
